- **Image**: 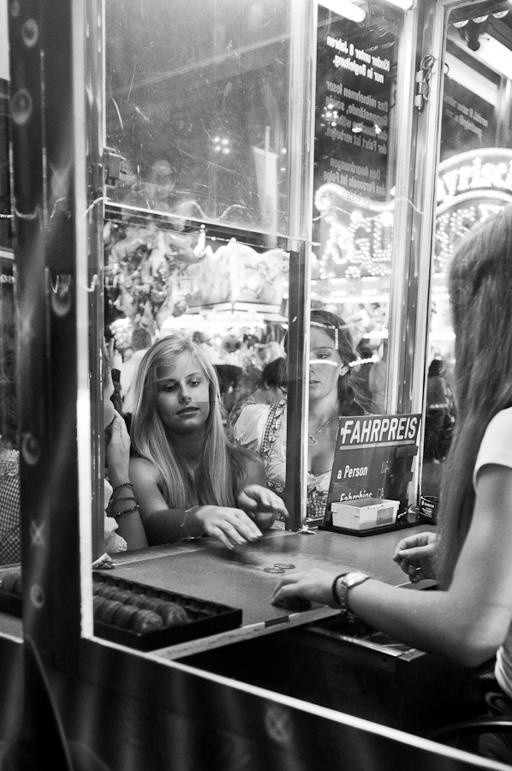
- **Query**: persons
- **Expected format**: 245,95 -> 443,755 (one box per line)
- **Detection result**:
270,198 -> 510,769
2,300 -> 455,555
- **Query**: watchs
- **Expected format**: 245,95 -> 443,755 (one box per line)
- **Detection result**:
338,569 -> 373,624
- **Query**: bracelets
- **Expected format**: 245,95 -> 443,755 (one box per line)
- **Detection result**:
332,573 -> 349,609
